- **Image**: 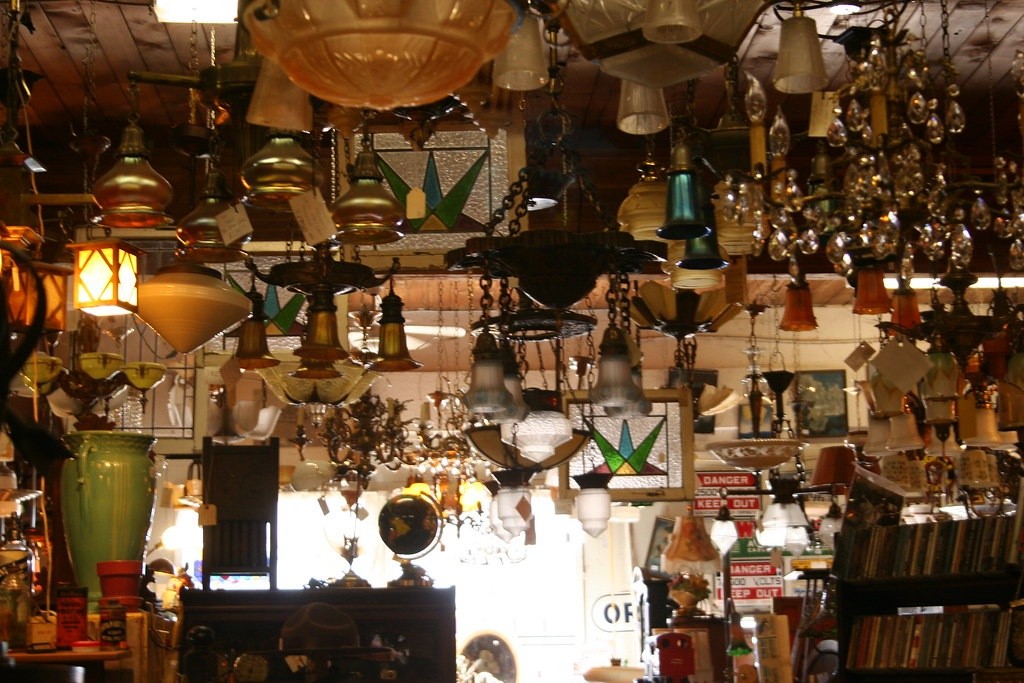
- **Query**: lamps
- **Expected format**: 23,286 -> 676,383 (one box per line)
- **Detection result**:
0,0 -> 1023,683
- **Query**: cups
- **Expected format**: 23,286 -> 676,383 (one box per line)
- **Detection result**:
97,559 -> 142,598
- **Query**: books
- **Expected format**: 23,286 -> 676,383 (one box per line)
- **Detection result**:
831,473 -> 1024,667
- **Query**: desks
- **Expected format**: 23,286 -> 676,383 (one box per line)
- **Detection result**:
6,646 -> 132,683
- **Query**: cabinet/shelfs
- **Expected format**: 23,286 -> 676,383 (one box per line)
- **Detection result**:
178,587 -> 457,683
832,514 -> 1024,683
670,618 -> 731,683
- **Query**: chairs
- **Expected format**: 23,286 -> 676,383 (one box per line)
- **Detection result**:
201,435 -> 281,590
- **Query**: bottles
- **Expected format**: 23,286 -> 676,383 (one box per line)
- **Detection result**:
0,527 -> 34,643
98,600 -> 127,651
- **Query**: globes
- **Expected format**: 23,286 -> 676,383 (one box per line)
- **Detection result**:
378,490 -> 444,589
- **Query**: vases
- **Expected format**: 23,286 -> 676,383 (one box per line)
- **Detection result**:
57,429 -> 157,613
94,560 -> 144,612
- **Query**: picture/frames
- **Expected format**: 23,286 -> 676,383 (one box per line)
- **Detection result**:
668,367 -> 717,434
739,402 -> 773,438
645,516 -> 675,582
795,369 -> 850,440
